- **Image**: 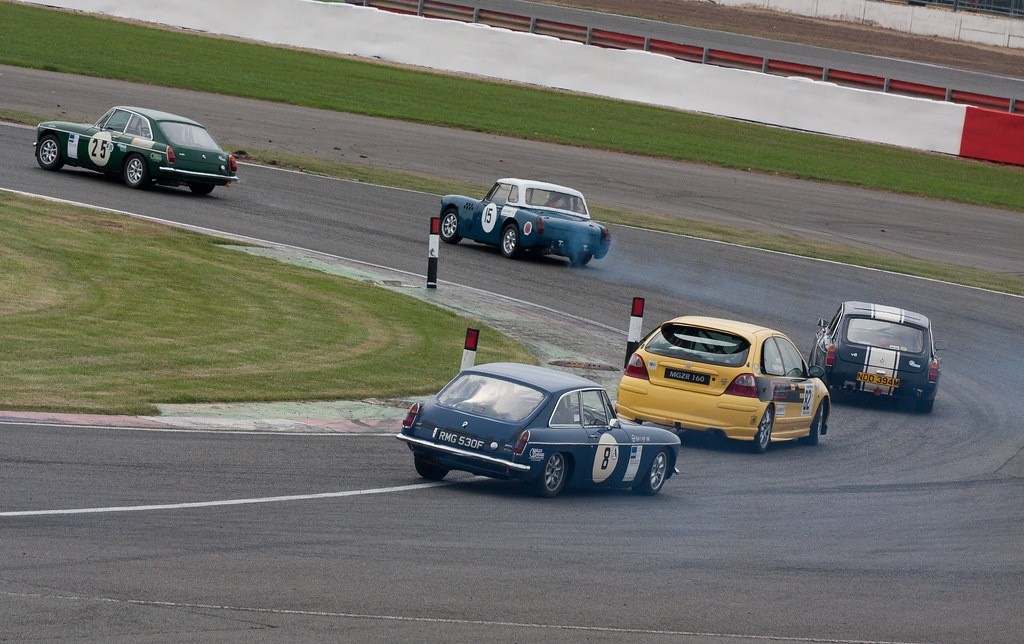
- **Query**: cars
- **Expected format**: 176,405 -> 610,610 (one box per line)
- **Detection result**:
808,298 -> 943,415
36,105 -> 239,197
396,362 -> 682,498
438,177 -> 611,269
613,315 -> 833,455
